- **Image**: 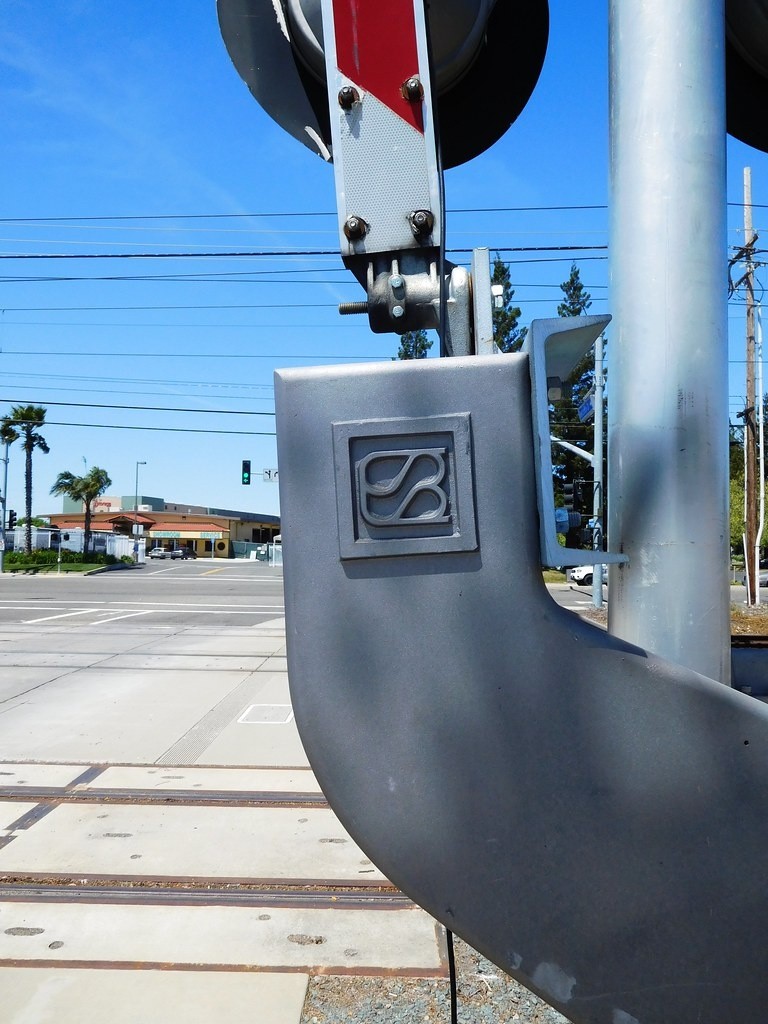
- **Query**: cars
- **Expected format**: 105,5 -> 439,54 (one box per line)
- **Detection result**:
760,558 -> 768,569
743,570 -> 768,587
149,548 -> 171,559
571,564 -> 608,587
171,548 -> 197,560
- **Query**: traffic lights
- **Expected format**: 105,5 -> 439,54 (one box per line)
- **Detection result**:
242,459 -> 250,485
565,478 -> 583,514
51,533 -> 59,540
64,535 -> 69,540
9,510 -> 17,529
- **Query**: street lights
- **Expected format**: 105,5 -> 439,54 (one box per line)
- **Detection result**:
136,461 -> 146,561
